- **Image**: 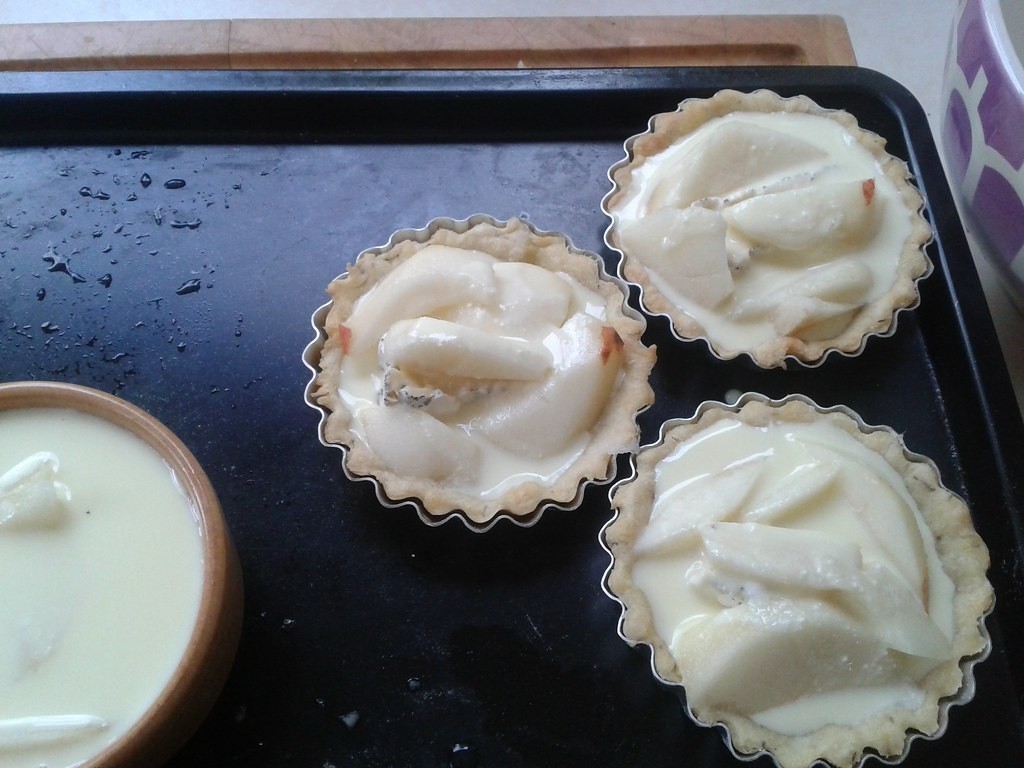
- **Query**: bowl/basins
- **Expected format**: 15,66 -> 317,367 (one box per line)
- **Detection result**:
0,379 -> 245,768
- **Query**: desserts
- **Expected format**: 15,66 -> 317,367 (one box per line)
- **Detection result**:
301,212 -> 658,533
600,88 -> 936,371
598,390 -> 997,768
